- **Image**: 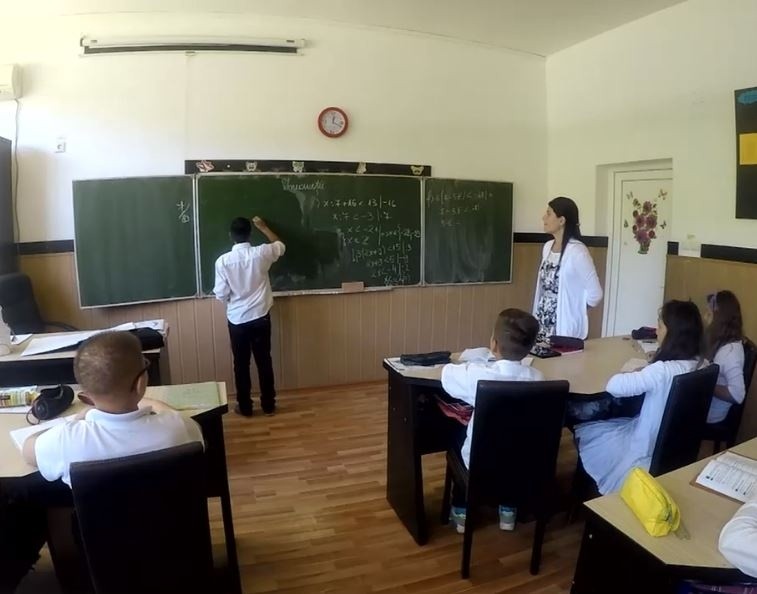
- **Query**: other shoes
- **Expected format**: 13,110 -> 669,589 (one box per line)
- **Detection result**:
499,504 -> 517,531
235,405 -> 253,416
265,409 -> 274,416
451,507 -> 480,533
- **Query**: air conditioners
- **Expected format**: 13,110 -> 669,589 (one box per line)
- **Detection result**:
0,63 -> 24,103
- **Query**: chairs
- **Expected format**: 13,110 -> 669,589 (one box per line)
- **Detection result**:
441,337 -> 757,580
0,273 -> 217,594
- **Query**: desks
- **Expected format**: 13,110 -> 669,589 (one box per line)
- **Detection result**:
569,438 -> 757,594
0,327 -> 173,387
382,335 -> 653,547
0,380 -> 242,594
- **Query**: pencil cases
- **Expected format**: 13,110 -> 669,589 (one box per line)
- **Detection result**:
400,351 -> 451,367
549,335 -> 584,351
632,327 -> 658,340
621,467 -> 681,536
26,386 -> 74,425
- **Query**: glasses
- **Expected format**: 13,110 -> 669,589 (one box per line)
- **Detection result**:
131,359 -> 151,394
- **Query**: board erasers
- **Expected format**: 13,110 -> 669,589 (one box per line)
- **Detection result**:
342,282 -> 364,290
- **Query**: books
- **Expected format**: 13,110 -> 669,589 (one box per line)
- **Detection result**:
549,346 -> 584,356
690,450 -> 757,501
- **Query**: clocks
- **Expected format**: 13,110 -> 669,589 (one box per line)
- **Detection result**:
318,107 -> 348,138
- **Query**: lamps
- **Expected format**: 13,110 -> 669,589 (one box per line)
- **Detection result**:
80,36 -> 306,57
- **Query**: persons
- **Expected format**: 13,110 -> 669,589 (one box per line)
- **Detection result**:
530,197 -> 603,347
680,485 -> 757,594
212,215 -> 286,417
441,308 -> 545,533
679,290 -> 746,435
573,299 -> 710,515
22,329 -> 207,594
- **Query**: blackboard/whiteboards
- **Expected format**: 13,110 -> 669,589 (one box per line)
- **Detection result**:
197,172 -> 423,298
72,174 -> 199,310
422,176 -> 514,285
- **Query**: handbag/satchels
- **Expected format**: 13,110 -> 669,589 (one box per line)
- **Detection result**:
129,327 -> 165,351
